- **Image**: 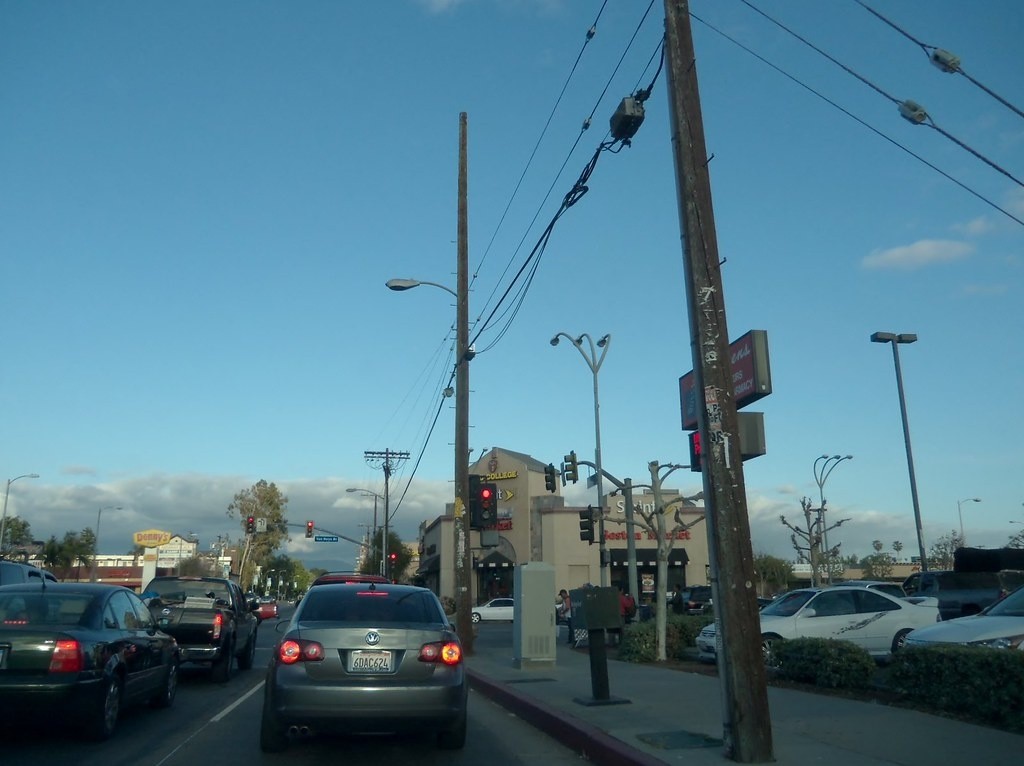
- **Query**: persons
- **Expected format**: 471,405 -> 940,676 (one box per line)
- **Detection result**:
617,585 -> 633,617
559,590 -> 574,642
667,584 -> 683,614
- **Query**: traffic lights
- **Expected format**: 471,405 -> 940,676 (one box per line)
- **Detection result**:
563,450 -> 579,484
688,431 -> 702,474
306,520 -> 313,540
544,463 -> 557,494
246,517 -> 255,535
479,483 -> 498,529
578,504 -> 596,546
390,554 -> 397,570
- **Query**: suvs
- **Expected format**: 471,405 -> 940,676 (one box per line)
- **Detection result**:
0,556 -> 63,623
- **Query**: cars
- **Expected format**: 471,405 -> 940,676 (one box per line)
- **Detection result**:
0,583 -> 184,742
472,598 -> 514,623
644,546 -> 1024,671
260,570 -> 469,753
245,592 -> 277,617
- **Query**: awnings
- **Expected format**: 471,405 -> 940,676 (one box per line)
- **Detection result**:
417,555 -> 439,573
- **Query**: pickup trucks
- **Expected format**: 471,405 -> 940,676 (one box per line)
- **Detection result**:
139,575 -> 261,684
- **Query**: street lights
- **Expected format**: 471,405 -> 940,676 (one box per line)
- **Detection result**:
813,453 -> 854,586
262,569 -> 277,592
385,277 -> 476,658
870,329 -> 930,574
957,497 -> 984,547
0,473 -> 42,553
89,505 -> 123,582
548,332 -> 612,586
344,487 -> 390,579
